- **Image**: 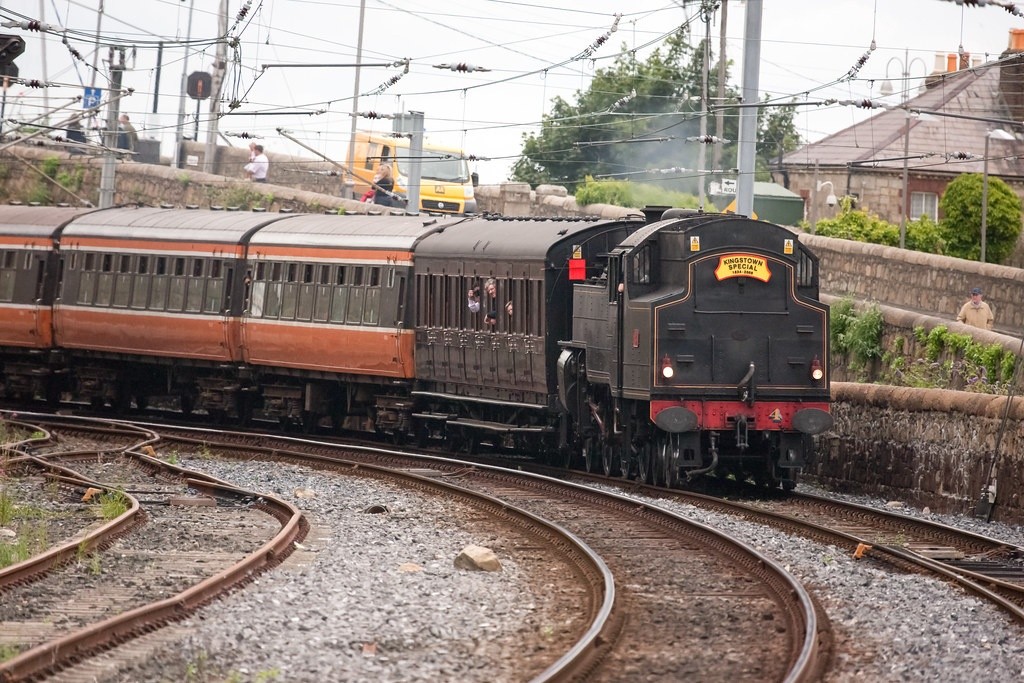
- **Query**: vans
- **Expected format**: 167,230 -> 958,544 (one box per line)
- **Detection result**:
340,129 -> 481,218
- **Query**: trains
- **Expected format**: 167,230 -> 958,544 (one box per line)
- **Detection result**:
1,195 -> 836,494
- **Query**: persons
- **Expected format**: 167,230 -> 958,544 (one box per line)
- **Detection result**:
485,278 -> 497,299
485,311 -> 496,325
467,280 -> 480,312
618,274 -> 650,293
66,113 -> 87,143
956,288 -> 994,331
116,114 -> 138,150
243,275 -> 251,287
363,164 -> 394,207
244,141 -> 269,183
505,300 -> 513,316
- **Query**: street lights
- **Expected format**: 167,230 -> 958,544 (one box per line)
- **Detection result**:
879,48 -> 934,107
900,111 -> 941,248
981,129 -> 1016,263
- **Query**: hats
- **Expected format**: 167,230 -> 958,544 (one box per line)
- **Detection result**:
971,288 -> 981,294
